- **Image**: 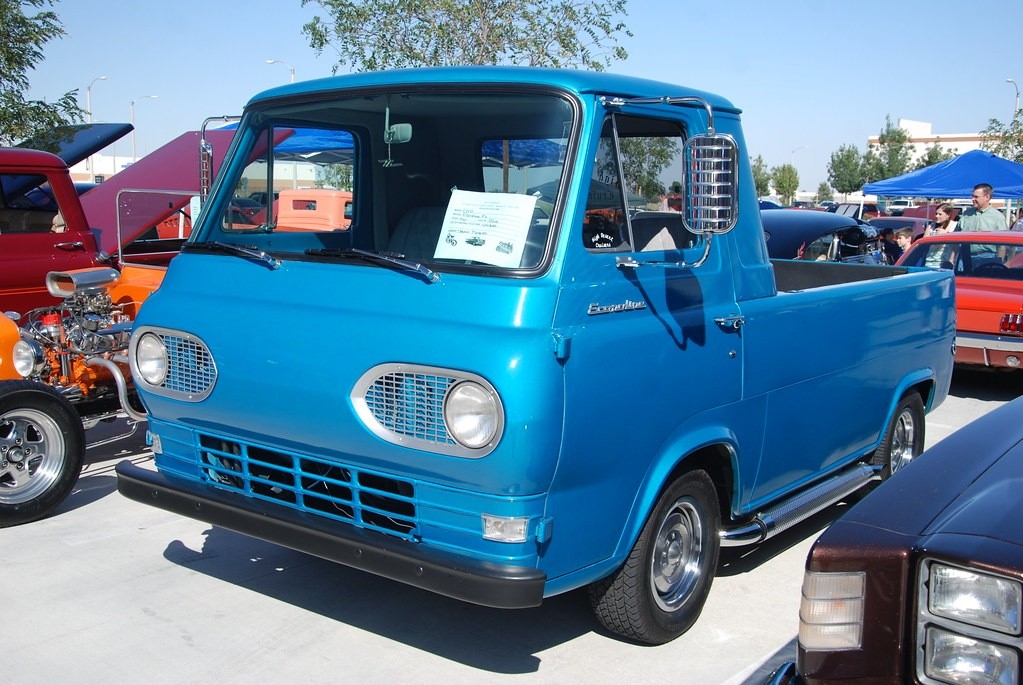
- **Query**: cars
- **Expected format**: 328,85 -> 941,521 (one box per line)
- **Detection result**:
895,230 -> 1023,370
534,199 -> 974,265
765,393 -> 1023,685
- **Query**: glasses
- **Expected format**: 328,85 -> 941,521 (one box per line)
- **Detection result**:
971,193 -> 987,199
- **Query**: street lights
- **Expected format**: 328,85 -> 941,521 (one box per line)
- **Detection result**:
266,59 -> 295,83
87,76 -> 107,184
1007,79 -> 1023,121
131,95 -> 159,164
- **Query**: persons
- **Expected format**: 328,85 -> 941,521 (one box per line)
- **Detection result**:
895,226 -> 925,267
880,228 -> 901,263
946,182 -> 1008,272
1009,208 -> 1023,252
922,203 -> 958,268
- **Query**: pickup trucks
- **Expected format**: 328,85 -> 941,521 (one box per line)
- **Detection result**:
116,67 -> 957,647
1,123 -> 353,527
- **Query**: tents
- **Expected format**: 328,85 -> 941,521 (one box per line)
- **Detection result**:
212,120 -> 567,191
857,150 -> 1023,230
526,176 -> 648,211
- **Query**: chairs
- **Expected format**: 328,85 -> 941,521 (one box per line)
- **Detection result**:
386,197 -> 450,263
